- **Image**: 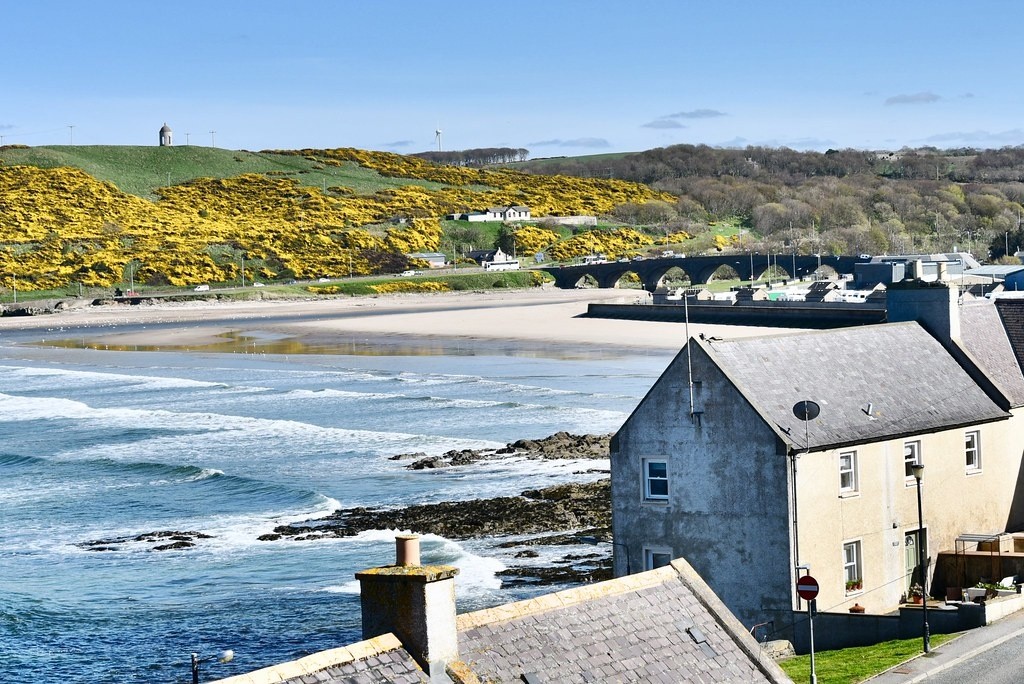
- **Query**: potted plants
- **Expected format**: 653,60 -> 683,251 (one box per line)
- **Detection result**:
845,577 -> 862,591
908,583 -> 929,604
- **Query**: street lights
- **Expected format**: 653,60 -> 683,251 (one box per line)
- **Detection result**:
910,464 -> 931,654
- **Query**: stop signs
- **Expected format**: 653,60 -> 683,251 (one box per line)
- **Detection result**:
796,575 -> 820,601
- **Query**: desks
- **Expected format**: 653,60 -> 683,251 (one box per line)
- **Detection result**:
968,586 -> 1017,602
